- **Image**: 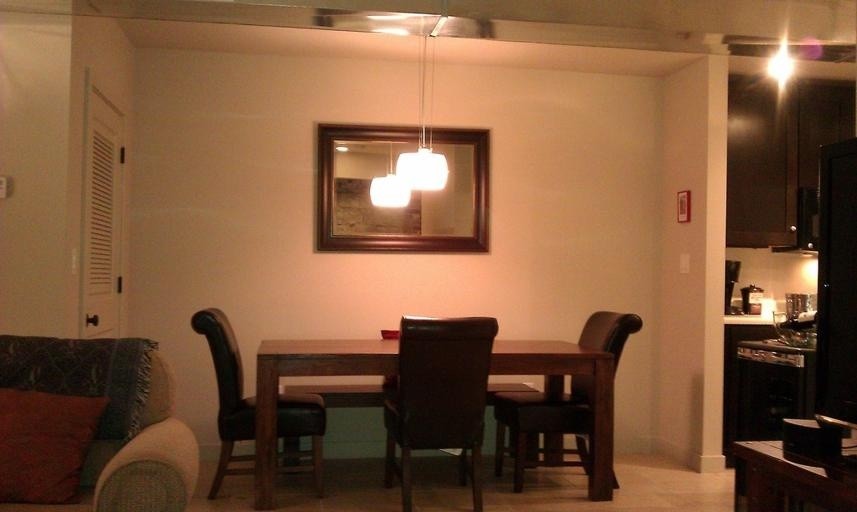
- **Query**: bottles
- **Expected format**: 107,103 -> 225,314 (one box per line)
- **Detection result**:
776,311 -> 818,329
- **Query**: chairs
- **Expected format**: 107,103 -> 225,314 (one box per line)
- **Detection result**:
495,310 -> 643,490
1,332 -> 197,511
190,306 -> 328,501
382,313 -> 496,511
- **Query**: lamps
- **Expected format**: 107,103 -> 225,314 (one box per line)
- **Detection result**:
369,142 -> 409,209
394,31 -> 449,191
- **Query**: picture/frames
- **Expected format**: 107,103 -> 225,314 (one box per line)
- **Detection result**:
676,190 -> 692,224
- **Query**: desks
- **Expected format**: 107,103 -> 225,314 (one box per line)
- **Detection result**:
254,340 -> 616,502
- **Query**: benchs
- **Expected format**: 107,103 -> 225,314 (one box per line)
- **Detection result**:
284,383 -> 541,469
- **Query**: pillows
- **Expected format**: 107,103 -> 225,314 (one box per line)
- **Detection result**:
0,388 -> 111,506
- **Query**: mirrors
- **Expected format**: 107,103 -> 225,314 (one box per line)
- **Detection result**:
314,120 -> 493,255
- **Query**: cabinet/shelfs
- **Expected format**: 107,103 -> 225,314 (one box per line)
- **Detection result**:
727,74 -> 800,247
730,438 -> 856,511
800,78 -> 856,188
